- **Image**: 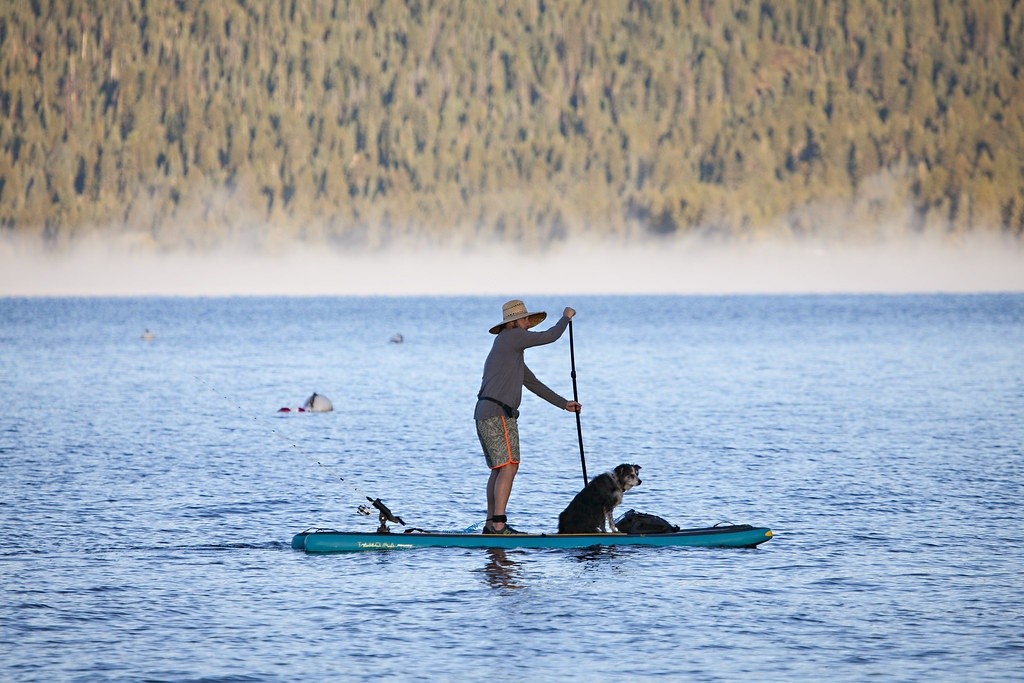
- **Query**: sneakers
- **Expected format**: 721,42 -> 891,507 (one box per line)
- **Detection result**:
490,524 -> 528,535
482,525 -> 493,534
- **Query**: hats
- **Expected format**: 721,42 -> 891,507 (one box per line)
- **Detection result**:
489,300 -> 548,334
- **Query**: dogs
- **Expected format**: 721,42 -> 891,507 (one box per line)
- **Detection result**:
556,463 -> 643,532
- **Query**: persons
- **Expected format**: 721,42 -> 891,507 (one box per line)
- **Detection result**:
473,299 -> 582,534
